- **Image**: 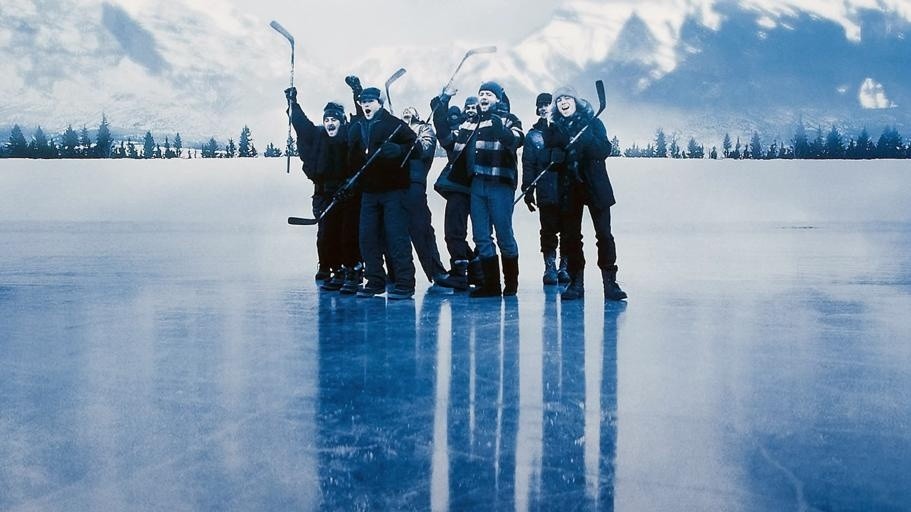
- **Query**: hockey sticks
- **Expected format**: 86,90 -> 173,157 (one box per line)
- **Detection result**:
270,20 -> 295,173
385,68 -> 406,115
400,45 -> 498,168
513,80 -> 607,205
287,126 -> 401,225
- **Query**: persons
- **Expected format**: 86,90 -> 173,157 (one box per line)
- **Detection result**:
522,92 -> 573,285
332,87 -> 422,300
386,106 -> 448,292
288,102 -> 356,280
318,174 -> 361,294
431,86 -> 511,291
544,85 -> 628,300
285,76 -> 361,280
454,82 -> 525,297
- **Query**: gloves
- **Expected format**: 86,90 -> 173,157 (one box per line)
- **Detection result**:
332,184 -> 349,201
578,121 -> 593,140
285,88 -> 297,104
431,98 -> 446,116
443,85 -> 456,95
524,192 -> 535,212
376,143 -> 400,159
345,76 -> 361,91
491,114 -> 505,137
551,148 -> 564,164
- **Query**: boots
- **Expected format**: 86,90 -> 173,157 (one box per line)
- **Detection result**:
340,261 -> 364,293
501,255 -> 519,296
468,260 -> 484,285
315,263 -> 330,280
543,258 -> 558,284
437,261 -> 469,291
561,273 -> 585,300
320,265 -> 345,290
558,258 -> 570,282
470,256 -> 502,298
602,267 -> 627,301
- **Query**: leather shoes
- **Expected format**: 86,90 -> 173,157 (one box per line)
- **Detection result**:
389,285 -> 415,298
356,285 -> 385,297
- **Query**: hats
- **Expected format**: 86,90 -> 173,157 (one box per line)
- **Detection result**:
358,88 -> 385,106
536,94 -> 552,115
465,97 -> 478,108
448,106 -> 461,117
479,82 -> 503,101
322,102 -> 345,125
554,86 -> 586,107
